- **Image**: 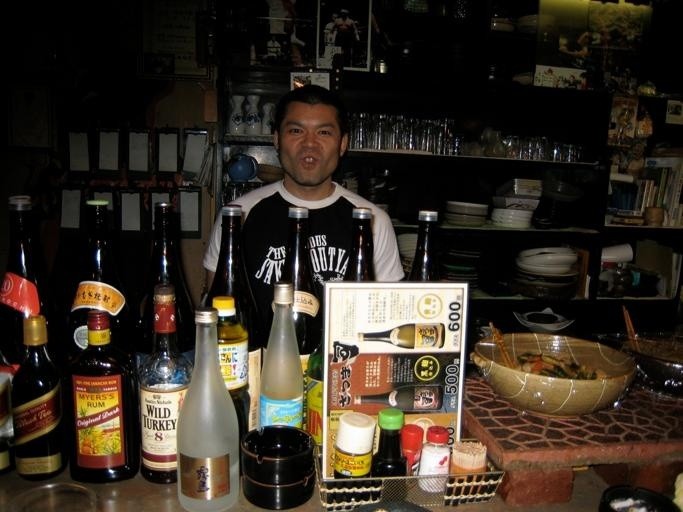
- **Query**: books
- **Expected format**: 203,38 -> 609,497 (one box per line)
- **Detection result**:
605,157 -> 682,227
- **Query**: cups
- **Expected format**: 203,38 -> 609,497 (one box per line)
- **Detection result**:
348,112 -> 577,164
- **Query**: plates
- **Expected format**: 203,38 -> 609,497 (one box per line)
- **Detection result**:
514,246 -> 578,278
444,201 -> 488,227
491,208 -> 533,229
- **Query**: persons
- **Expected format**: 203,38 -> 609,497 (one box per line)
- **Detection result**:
203,85 -> 405,354
325,15 -> 338,47
329,10 -> 360,57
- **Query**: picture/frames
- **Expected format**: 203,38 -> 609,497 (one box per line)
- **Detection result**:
316,0 -> 370,73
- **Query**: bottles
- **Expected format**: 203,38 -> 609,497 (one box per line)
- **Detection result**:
399,423 -> 423,487
61,310 -> 140,482
333,412 -> 375,504
0,196 -> 54,372
260,281 -> 303,429
262,208 -> 321,432
68,199 -> 132,349
355,386 -> 442,411
341,208 -> 375,282
406,211 -> 444,283
176,309 -> 239,511
418,428 -> 449,492
141,286 -> 194,483
137,203 -> 195,356
373,409 -> 407,500
360,323 -> 445,349
11,316 -> 64,481
202,205 -> 262,432
213,297 -> 250,477
306,336 -> 324,455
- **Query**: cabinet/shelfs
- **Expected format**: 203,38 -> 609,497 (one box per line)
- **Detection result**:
218,60 -> 683,337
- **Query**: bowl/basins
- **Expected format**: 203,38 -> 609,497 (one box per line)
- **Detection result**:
467,331 -> 643,424
